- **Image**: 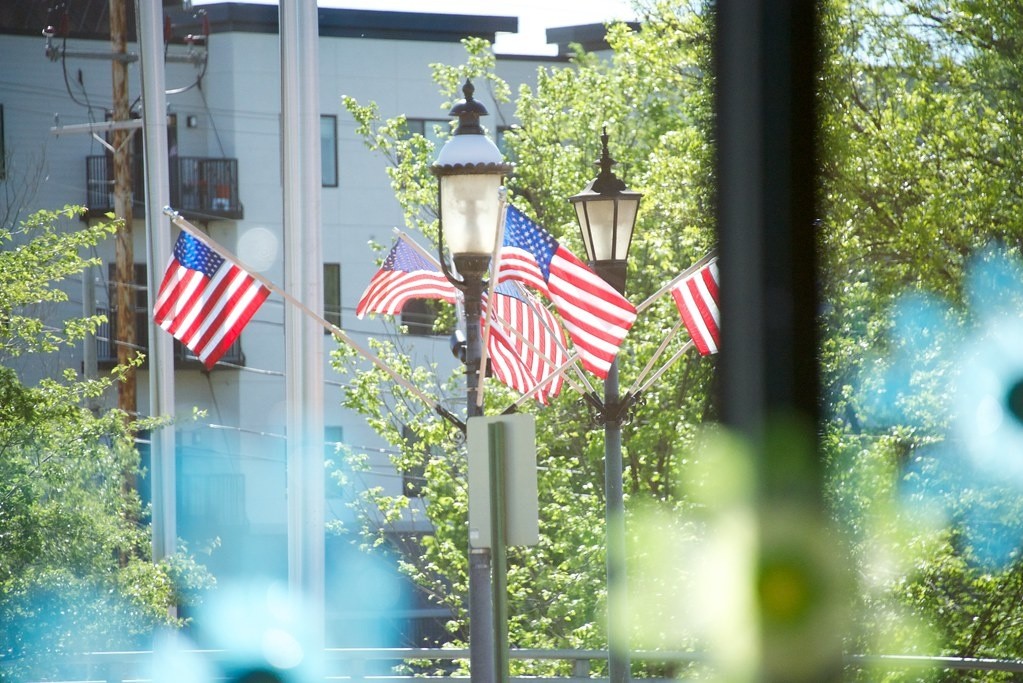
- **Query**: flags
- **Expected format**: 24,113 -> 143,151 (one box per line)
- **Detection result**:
497,203 -> 638,380
153,229 -> 271,372
478,259 -> 568,404
670,259 -> 721,356
355,237 -> 457,320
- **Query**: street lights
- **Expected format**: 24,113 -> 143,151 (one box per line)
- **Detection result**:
569,126 -> 644,683
430,77 -> 510,682
